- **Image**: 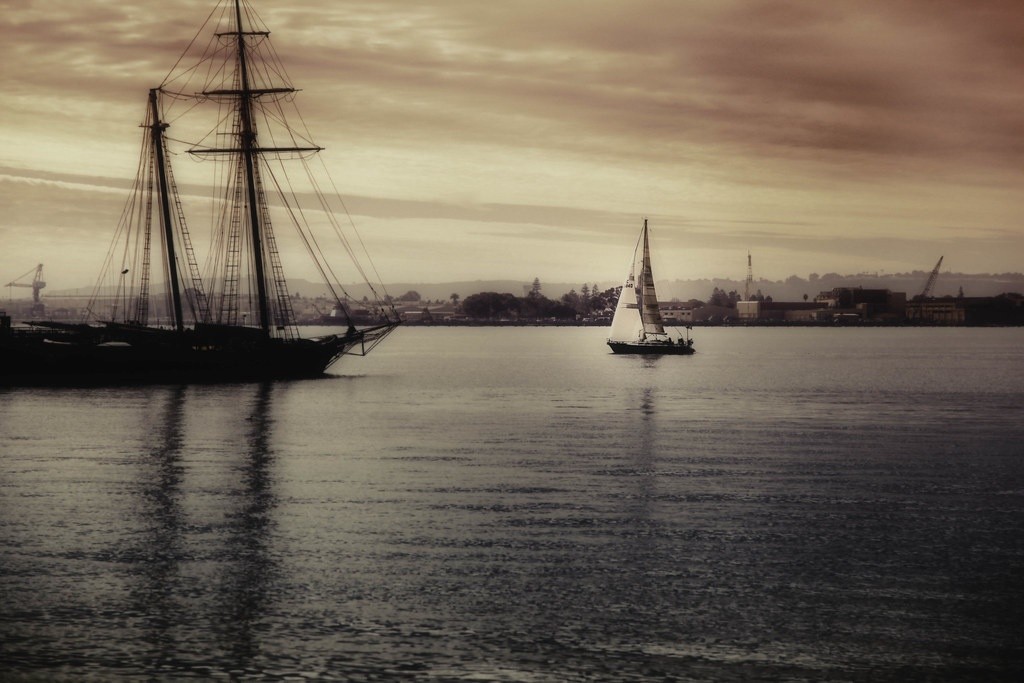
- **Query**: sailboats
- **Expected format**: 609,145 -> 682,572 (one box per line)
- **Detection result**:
605,219 -> 695,356
0,0 -> 408,375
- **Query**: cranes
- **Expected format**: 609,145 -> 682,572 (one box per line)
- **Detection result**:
5,264 -> 46,304
